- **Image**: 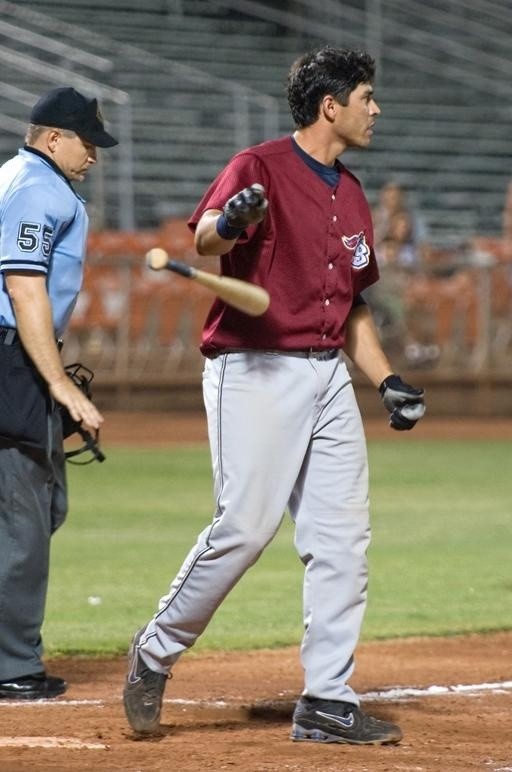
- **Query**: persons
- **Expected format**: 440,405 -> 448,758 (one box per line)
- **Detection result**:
1,85 -> 123,704
122,45 -> 429,745
361,178 -> 446,370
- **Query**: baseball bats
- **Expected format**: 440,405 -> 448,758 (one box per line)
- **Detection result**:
144,245 -> 270,319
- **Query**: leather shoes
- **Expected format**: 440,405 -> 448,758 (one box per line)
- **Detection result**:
0,675 -> 68,700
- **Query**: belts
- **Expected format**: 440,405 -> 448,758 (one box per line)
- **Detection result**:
217,346 -> 339,361
0,326 -> 63,353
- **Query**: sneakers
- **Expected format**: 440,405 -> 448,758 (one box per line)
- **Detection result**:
123,627 -> 173,735
288,695 -> 404,746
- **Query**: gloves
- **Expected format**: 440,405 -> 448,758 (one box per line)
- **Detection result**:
379,374 -> 427,431
217,182 -> 269,240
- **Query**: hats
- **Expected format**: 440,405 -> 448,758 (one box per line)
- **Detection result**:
29,87 -> 118,148
